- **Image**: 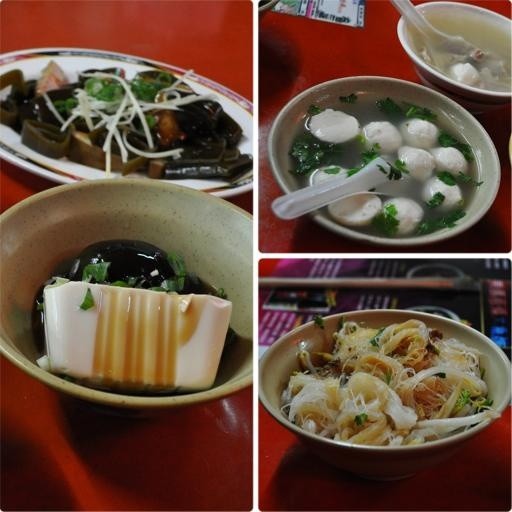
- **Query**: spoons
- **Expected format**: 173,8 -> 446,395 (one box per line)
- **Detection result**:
389,1 -> 478,74
271,154 -> 414,220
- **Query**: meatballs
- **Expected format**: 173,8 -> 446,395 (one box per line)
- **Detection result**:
309,108 -> 468,236
450,62 -> 481,86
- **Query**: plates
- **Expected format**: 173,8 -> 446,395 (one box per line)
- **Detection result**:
1,48 -> 253,199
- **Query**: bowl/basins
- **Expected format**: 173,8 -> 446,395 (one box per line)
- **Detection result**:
396,1 -> 512,114
267,75 -> 502,247
0,179 -> 253,412
259,309 -> 511,481
